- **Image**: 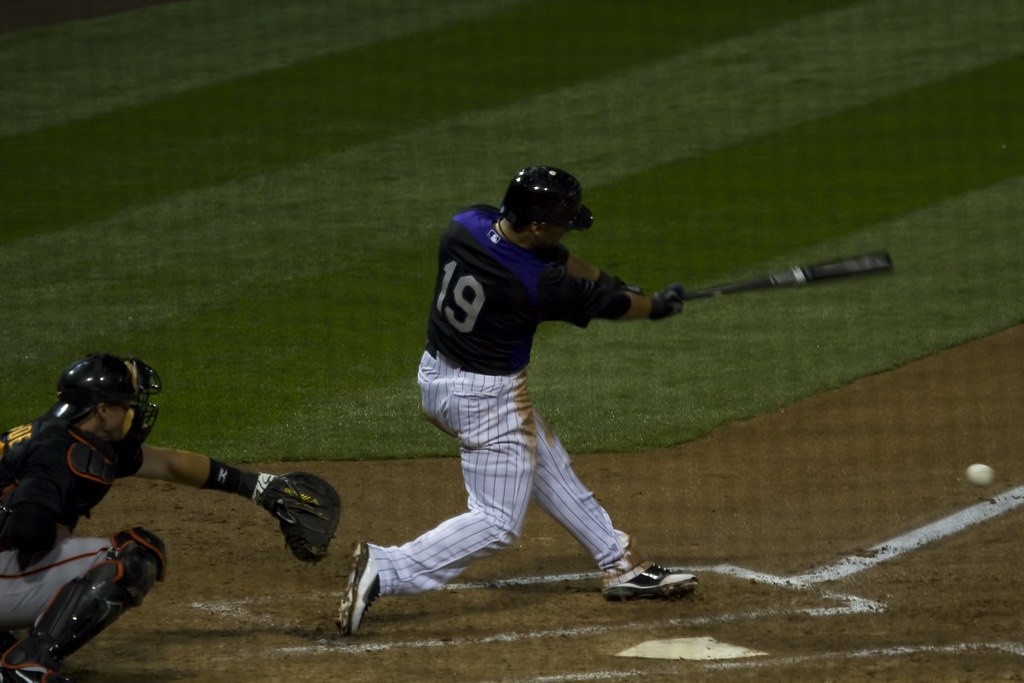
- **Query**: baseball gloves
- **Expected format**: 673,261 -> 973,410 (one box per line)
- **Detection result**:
258,472 -> 341,562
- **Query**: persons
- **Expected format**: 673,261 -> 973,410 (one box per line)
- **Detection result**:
0,352 -> 342,683
336,165 -> 699,637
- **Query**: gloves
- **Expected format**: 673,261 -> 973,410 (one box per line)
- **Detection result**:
649,284 -> 686,318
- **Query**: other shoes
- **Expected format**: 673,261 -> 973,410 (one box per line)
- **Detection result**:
0,667 -> 45,683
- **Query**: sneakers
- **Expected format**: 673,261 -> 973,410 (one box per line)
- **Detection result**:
603,563 -> 700,602
337,541 -> 380,636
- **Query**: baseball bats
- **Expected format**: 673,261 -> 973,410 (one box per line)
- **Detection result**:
681,251 -> 893,301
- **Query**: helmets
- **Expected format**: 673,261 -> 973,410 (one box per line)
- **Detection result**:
46,353 -> 136,421
499,164 -> 593,230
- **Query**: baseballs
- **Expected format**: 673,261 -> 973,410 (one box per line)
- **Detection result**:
966,463 -> 994,486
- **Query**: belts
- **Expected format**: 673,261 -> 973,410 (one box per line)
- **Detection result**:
427,343 -> 481,374
